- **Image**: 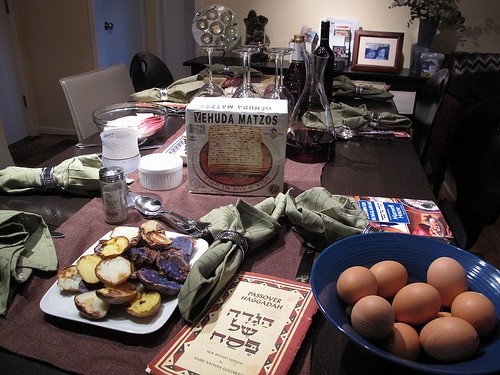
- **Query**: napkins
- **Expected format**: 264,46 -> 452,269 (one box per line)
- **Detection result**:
332,75 -> 395,101
300,100 -> 412,131
129,64 -> 263,103
178,187 -> 384,325
0,209 -> 59,315
0,154 -> 134,194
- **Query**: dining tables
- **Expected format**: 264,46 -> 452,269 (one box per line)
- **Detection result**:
0,78 -> 456,375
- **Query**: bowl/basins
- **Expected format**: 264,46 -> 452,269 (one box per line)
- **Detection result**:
311,232 -> 500,375
92,101 -> 169,146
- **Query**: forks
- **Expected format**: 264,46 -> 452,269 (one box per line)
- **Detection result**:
76,137 -> 147,148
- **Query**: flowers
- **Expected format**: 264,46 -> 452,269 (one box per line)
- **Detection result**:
388,0 -> 467,48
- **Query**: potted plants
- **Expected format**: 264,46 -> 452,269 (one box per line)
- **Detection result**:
244,10 -> 268,47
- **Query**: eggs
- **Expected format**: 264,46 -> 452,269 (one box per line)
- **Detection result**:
337,256 -> 495,360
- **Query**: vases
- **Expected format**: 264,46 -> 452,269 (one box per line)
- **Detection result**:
410,17 -> 439,74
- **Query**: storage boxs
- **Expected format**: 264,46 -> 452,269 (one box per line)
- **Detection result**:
185,96 -> 288,196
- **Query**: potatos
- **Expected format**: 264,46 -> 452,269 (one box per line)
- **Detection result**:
55,220 -> 172,319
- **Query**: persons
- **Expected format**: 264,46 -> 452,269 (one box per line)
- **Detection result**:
334,48 -> 346,57
365,47 -> 387,60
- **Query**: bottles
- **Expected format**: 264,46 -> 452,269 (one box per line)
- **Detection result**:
99,166 -> 128,224
311,20 -> 335,111
286,42 -> 337,164
99,128 -> 141,175
284,34 -> 309,120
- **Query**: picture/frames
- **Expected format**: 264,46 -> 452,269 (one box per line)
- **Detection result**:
351,30 -> 405,74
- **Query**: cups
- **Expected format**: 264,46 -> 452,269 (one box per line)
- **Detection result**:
420,52 -> 445,78
138,152 -> 183,191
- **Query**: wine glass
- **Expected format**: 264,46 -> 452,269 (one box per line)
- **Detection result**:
199,42 -> 295,119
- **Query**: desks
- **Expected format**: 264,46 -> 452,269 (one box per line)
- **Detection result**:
182,57 -> 430,95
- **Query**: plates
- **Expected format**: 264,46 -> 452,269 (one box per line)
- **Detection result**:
39,226 -> 209,335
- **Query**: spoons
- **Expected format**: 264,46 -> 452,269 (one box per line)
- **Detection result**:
134,197 -> 211,238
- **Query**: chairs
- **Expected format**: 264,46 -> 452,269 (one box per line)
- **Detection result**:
59,53 -> 174,142
413,69 -> 460,199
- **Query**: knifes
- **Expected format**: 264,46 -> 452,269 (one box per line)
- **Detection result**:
94,145 -> 160,156
50,232 -> 65,238
295,247 -> 316,283
